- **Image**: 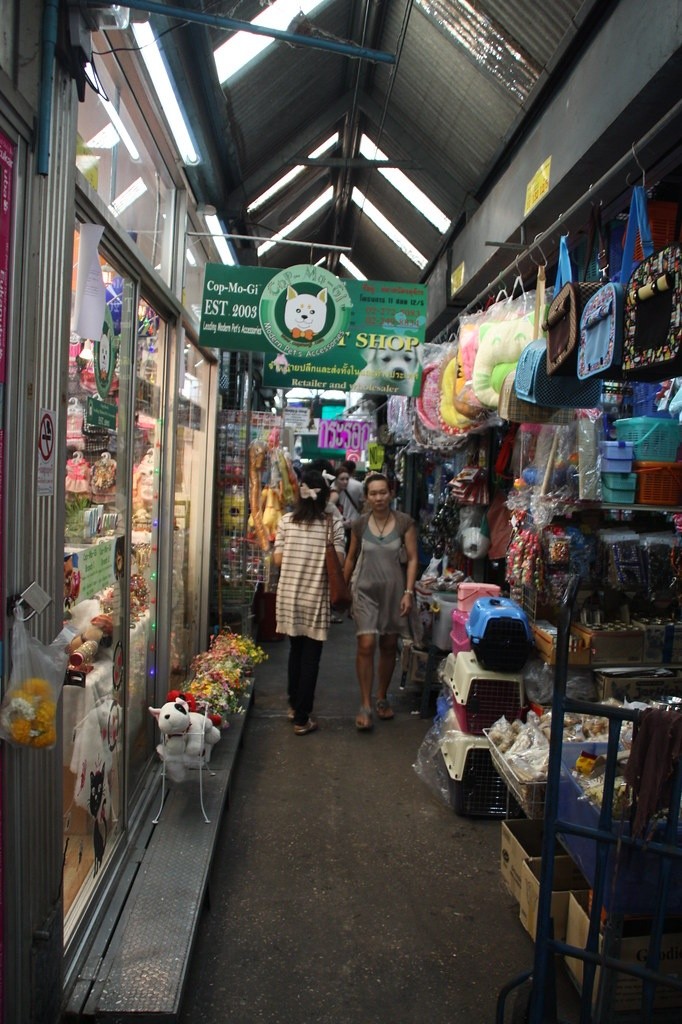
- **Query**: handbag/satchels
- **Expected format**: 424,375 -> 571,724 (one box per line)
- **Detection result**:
448,432 -> 492,505
0,602 -> 84,748
324,514 -> 354,612
499,244 -> 682,426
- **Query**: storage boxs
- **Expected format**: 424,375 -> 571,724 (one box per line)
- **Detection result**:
556,742 -> 682,916
633,619 -> 682,664
501,819 -> 568,903
457,582 -> 500,612
564,889 -> 682,1024
634,461 -> 682,505
600,441 -> 636,473
613,416 -> 682,462
519,855 -> 589,943
581,623 -> 644,664
529,620 -> 591,665
430,593 -> 458,650
602,473 -> 638,504
595,671 -> 682,702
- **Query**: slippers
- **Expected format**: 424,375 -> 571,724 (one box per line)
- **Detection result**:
296,718 -> 317,734
375,701 -> 395,721
355,705 -> 374,731
287,706 -> 297,720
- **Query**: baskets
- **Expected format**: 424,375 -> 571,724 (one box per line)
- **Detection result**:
482,727 -> 548,818
632,463 -> 682,504
614,416 -> 682,462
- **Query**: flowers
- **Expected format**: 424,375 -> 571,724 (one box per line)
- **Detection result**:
177,625 -> 266,728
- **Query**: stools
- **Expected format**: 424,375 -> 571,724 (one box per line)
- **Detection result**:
421,648 -> 449,715
400,644 -> 428,686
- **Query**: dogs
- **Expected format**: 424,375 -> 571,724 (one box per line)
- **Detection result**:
349,336 -> 424,397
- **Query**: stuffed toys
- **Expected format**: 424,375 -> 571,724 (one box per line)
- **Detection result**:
148,690 -> 221,757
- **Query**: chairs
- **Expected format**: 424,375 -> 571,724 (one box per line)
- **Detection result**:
153,732 -> 215,824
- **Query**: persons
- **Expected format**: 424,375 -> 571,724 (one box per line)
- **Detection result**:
343,474 -> 417,730
325,461 -> 363,625
272,469 -> 347,735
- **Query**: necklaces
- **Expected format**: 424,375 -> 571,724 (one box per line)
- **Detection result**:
370,511 -> 391,538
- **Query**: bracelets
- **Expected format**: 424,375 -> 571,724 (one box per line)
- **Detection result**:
403,589 -> 414,594
